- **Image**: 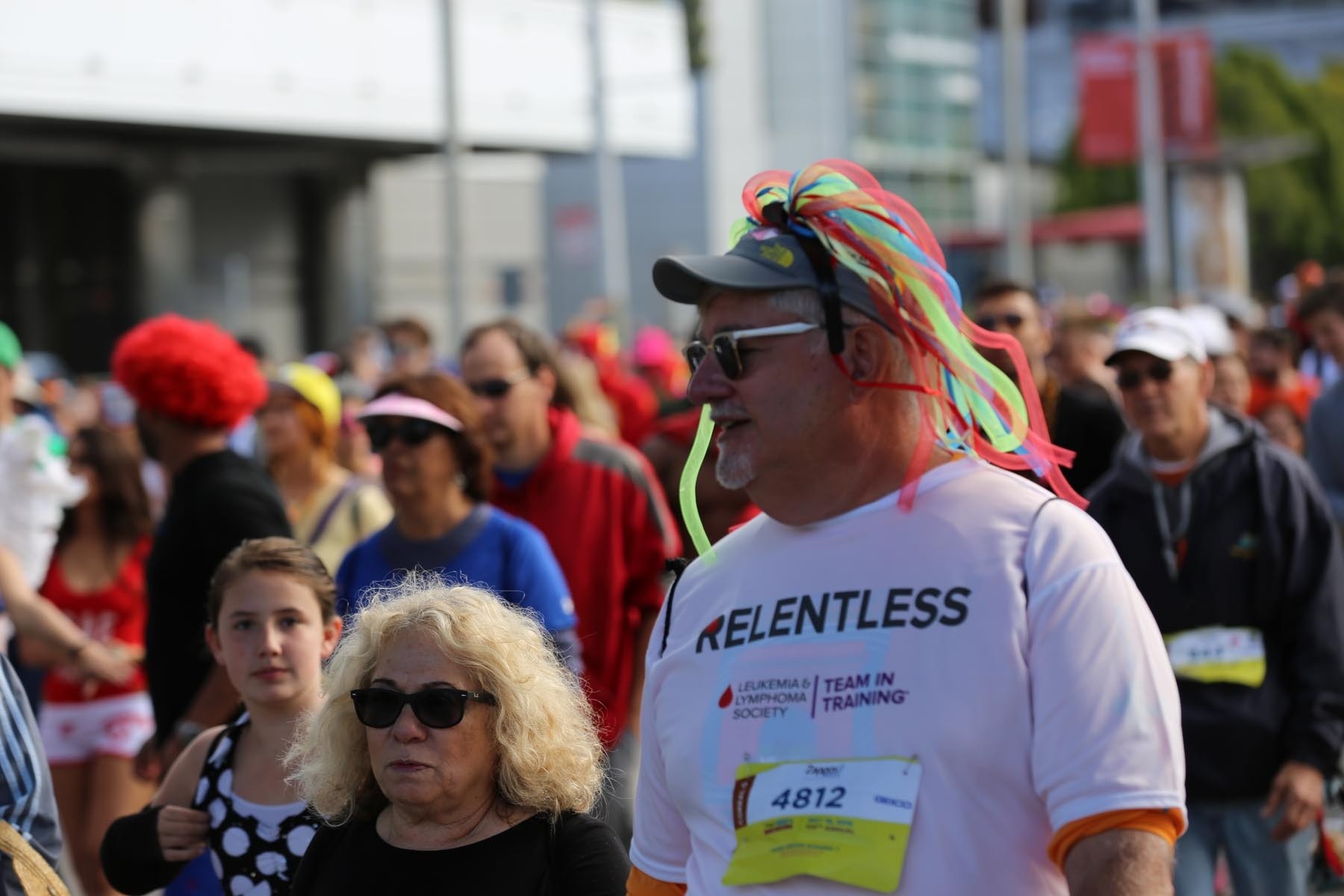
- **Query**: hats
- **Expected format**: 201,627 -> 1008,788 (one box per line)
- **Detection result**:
356,391 -> 464,430
0,323 -> 23,371
1182,305 -> 1236,354
267,363 -> 342,430
652,160 -> 914,338
111,313 -> 269,429
1104,306 -> 1207,364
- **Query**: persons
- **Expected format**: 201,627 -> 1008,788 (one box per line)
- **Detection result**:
0,262 -> 1344,896
608,163 -> 1189,896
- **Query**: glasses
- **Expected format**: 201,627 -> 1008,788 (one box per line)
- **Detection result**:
367,424 -> 443,451
467,367 -> 533,402
350,688 -> 498,729
974,315 -> 1024,329
680,322 -> 823,380
1117,364 -> 1172,391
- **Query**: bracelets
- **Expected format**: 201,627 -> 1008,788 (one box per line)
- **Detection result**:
68,633 -> 92,657
